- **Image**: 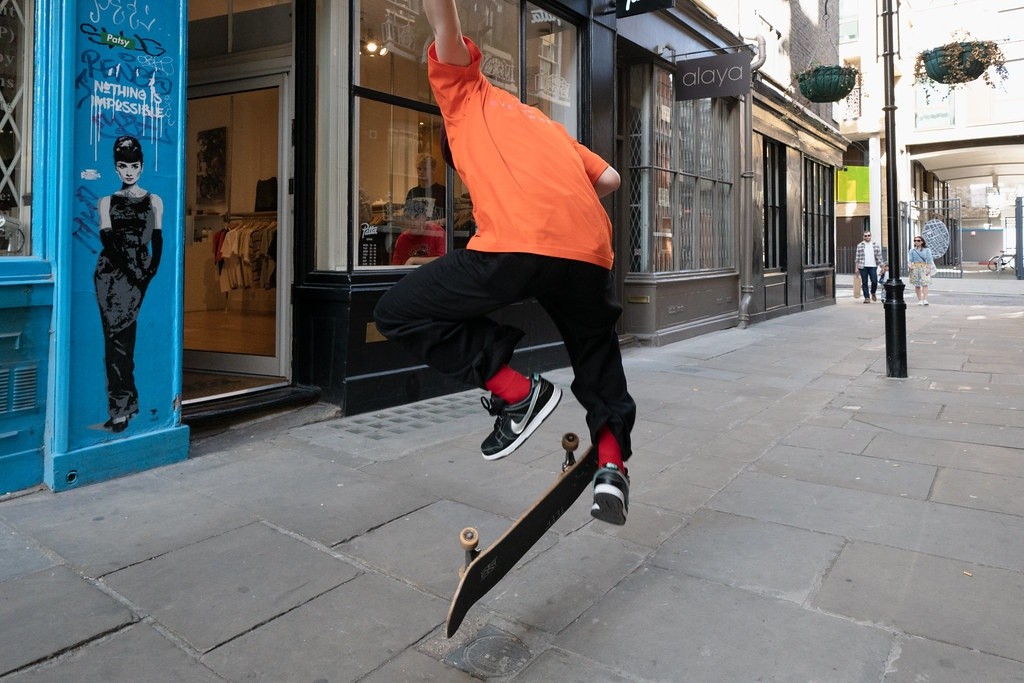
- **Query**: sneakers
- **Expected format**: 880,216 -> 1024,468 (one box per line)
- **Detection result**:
590,460 -> 630,526
481,372 -> 563,462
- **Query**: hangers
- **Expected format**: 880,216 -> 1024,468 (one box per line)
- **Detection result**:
370,215 -> 384,224
224,216 -> 277,230
454,212 -> 474,228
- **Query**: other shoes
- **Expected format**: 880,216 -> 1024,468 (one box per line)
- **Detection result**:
870,290 -> 877,301
863,298 -> 870,303
918,300 -> 923,305
923,300 -> 929,305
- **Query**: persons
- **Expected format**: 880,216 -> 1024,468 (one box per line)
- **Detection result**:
879,261 -> 890,304
372,2 -> 636,527
392,200 -> 447,264
908,235 -> 932,305
854,230 -> 886,304
371,153 -> 448,226
93,136 -> 164,433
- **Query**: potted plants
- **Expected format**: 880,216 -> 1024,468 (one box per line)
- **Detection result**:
781,61 -> 868,121
912,33 -> 1009,105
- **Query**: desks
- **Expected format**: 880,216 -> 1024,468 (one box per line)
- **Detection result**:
375,225 -> 469,252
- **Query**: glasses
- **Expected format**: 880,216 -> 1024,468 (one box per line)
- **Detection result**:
865,235 -> 870,237
914,239 -> 921,242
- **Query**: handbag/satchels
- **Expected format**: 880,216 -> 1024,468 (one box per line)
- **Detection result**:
853,273 -> 861,298
928,258 -> 937,276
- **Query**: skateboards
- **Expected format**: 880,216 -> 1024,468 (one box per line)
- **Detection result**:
445,431 -> 596,641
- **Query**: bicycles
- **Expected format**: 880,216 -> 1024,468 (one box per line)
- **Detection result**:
988,248 -> 1016,272
